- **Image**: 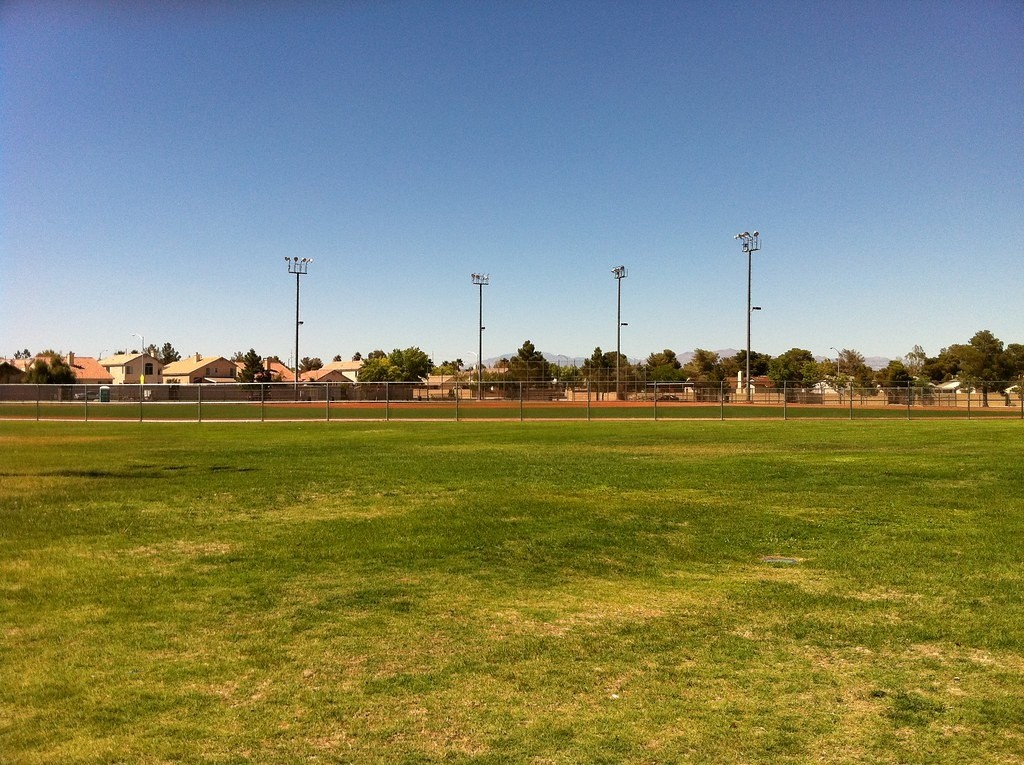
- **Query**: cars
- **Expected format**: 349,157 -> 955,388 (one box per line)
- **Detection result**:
247,393 -> 272,401
75,391 -> 97,399
1005,385 -> 1021,393
657,395 -> 679,401
802,382 -> 844,396
548,393 -> 567,401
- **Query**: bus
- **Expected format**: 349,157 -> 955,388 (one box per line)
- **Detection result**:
98,386 -> 110,402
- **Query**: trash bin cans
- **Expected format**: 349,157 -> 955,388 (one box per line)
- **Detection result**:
724,396 -> 729,402
330,397 -> 334,404
99,386 -> 110,402
418,396 -> 422,402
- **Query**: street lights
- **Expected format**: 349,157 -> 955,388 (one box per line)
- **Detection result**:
830,346 -> 839,378
471,273 -> 490,400
611,265 -> 628,393
734,231 -> 761,402
284,257 -> 314,401
100,350 -> 108,358
132,334 -> 144,384
